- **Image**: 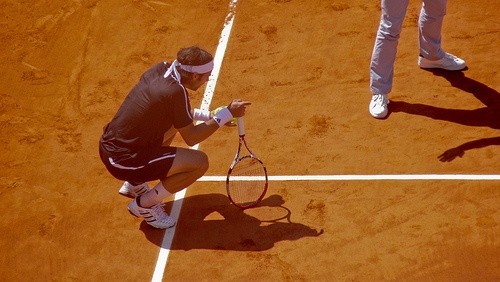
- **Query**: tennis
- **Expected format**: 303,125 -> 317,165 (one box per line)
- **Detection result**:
213,107 -> 223,116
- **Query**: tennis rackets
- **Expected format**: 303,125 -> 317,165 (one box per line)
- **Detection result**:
226,115 -> 269,209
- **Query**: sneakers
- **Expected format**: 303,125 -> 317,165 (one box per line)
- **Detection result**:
118,180 -> 150,200
369,93 -> 390,118
418,51 -> 466,70
127,196 -> 176,229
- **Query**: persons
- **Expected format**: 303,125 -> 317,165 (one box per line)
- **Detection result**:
368,0 -> 466,119
98,46 -> 252,230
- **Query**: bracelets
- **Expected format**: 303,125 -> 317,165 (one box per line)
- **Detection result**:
194,109 -> 210,122
214,108 -> 233,128
